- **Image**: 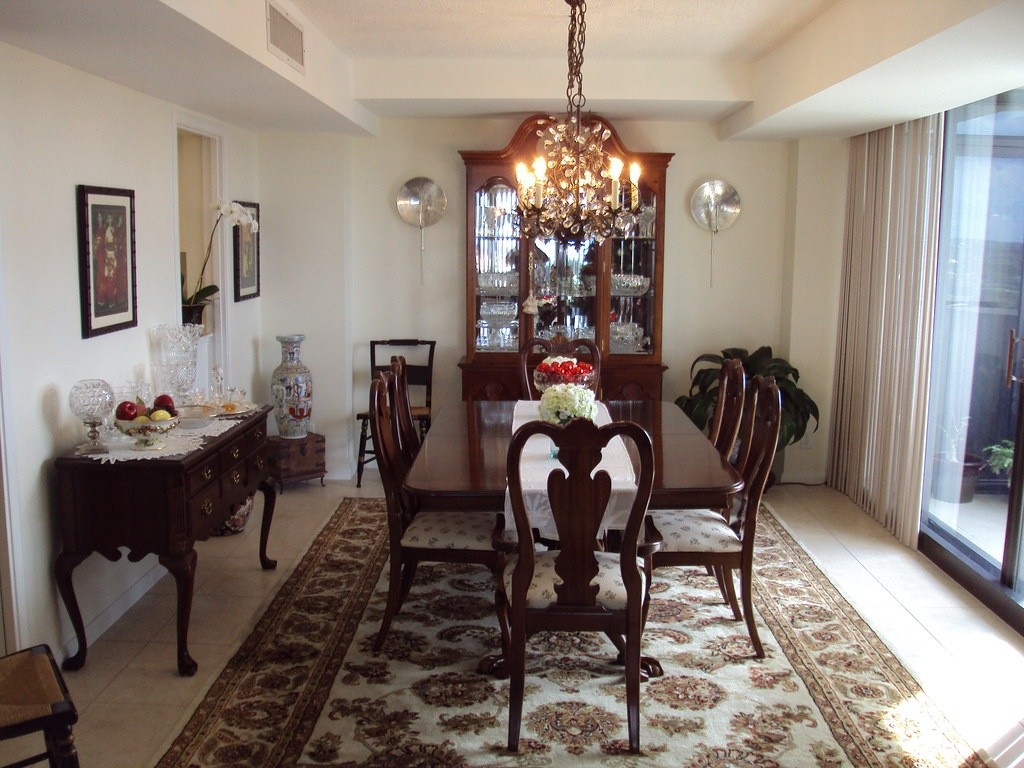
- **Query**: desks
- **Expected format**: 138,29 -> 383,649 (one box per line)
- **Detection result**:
53,405 -> 284,678
408,401 -> 743,677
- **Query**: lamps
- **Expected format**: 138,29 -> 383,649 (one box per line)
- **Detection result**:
513,1 -> 644,244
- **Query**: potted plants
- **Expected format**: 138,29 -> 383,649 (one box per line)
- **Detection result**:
677,345 -> 817,462
979,442 -> 1014,487
934,403 -> 984,505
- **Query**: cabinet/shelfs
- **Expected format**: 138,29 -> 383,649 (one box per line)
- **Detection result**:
458,116 -> 675,486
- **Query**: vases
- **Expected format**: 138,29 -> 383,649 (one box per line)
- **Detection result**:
548,432 -> 564,456
181,299 -> 209,332
271,333 -> 314,439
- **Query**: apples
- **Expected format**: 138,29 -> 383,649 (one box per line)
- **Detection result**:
115,401 -> 138,421
153,395 -> 175,415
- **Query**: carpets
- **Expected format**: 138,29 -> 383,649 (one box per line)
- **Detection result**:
151,497 -> 1000,767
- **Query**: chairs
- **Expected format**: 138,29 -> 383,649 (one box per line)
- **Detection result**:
623,377 -> 786,658
521,331 -> 601,397
0,643 -> 81,768
369,378 -> 511,664
652,356 -> 749,510
353,339 -> 436,489
494,421 -> 659,753
387,353 -> 421,461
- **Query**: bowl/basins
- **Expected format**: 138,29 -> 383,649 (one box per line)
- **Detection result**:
480,302 -> 517,327
612,274 -> 650,295
478,273 -> 519,298
177,406 -> 217,429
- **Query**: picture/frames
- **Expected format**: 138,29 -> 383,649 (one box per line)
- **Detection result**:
230,203 -> 261,302
75,186 -> 140,338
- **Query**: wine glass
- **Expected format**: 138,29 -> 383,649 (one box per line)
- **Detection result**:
638,205 -> 656,237
194,385 -> 247,411
476,327 -> 518,352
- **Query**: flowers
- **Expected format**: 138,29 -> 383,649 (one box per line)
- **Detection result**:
539,385 -> 598,427
185,197 -> 262,297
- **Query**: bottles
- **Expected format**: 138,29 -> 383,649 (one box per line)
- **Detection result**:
271,335 -> 313,440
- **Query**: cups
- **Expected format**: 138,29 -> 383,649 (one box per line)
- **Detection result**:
536,325 -> 595,353
609,321 -> 651,352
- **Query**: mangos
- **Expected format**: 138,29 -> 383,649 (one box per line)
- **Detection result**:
131,405 -> 172,422
